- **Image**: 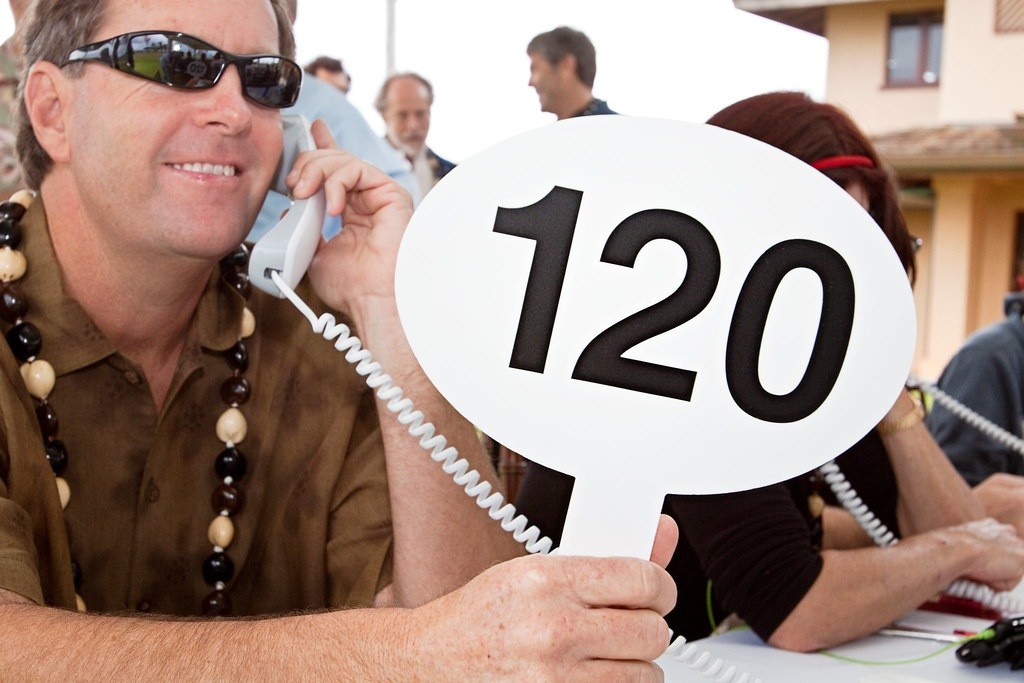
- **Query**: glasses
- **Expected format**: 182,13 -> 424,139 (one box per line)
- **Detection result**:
55,28 -> 303,109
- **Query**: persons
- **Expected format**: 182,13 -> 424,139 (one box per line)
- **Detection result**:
304,57 -> 352,95
897,295 -> 1024,539
371,72 -> 458,185
524,29 -> 617,120
0,0 -> 680,683
515,92 -> 1024,653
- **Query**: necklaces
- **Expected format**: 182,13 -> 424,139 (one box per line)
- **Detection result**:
0,190 -> 257,622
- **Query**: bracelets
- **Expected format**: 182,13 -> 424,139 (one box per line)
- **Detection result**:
876,403 -> 924,438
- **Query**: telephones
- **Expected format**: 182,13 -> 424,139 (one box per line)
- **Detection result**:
247,112 -> 326,301
